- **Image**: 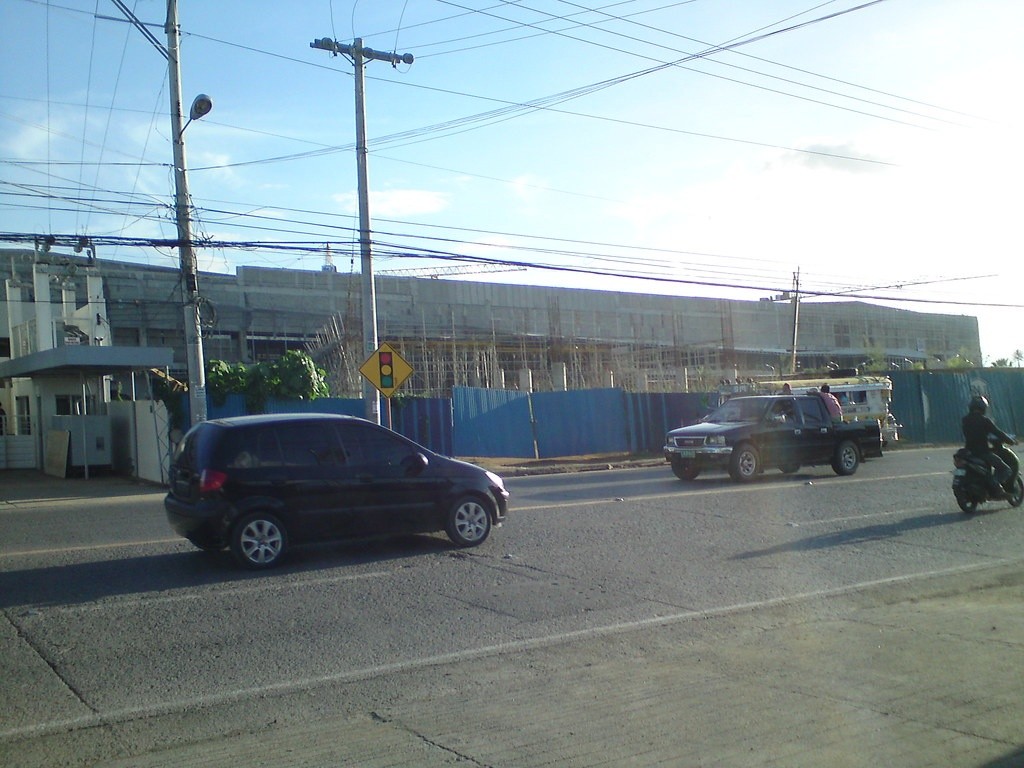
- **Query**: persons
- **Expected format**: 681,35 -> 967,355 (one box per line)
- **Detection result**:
777,383 -> 794,395
806,385 -> 849,423
962,396 -> 1019,491
771,402 -> 794,423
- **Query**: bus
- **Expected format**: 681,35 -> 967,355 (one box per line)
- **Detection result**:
716,377 -> 903,449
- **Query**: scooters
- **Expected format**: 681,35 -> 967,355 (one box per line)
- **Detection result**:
950,433 -> 1024,513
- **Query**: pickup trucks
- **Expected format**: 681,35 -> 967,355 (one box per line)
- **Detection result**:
662,395 -> 883,483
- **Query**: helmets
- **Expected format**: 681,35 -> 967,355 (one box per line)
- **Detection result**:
970,396 -> 990,416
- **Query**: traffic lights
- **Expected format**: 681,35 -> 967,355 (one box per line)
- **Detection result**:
378,351 -> 393,388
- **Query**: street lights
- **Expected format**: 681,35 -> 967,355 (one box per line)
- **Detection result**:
172,94 -> 215,427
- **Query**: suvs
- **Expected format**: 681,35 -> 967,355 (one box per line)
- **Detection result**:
163,414 -> 509,570
